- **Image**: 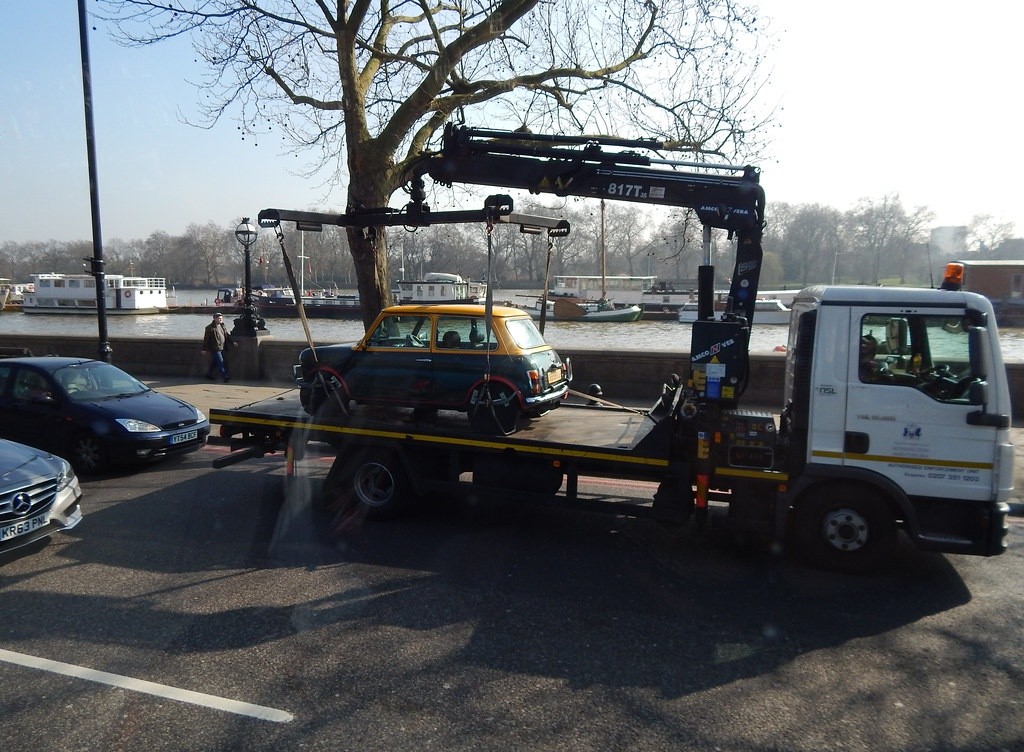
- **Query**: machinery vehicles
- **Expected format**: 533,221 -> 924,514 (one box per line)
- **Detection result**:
206,270 -> 1015,567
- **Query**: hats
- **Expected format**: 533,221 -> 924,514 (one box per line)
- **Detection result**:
212,313 -> 223,318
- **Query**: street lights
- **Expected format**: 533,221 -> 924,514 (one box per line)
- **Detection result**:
230,215 -> 270,339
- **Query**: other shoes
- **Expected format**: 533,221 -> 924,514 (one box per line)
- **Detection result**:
205,375 -> 216,381
224,377 -> 229,383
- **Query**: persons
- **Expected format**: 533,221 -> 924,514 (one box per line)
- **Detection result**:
201,313 -> 238,383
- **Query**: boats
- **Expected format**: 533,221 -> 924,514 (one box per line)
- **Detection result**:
529,301 -> 642,323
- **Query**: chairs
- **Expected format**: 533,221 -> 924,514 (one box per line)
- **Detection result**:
23,375 -> 46,397
464,329 -> 485,349
63,371 -> 81,394
443,331 -> 461,349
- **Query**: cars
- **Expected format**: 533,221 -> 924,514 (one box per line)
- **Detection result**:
292,299 -> 574,438
0,436 -> 83,555
0,354 -> 211,472
